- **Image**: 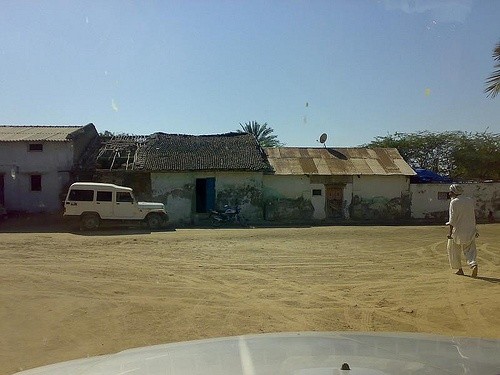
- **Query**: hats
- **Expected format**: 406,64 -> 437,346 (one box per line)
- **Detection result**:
449,183 -> 464,196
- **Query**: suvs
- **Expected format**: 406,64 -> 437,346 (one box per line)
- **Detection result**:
61,182 -> 169,232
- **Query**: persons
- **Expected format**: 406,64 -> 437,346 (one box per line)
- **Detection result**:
446,184 -> 479,278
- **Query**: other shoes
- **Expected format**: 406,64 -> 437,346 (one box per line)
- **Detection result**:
454,271 -> 464,275
472,266 -> 479,277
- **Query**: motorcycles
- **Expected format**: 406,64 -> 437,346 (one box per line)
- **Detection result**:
209,204 -> 241,227
